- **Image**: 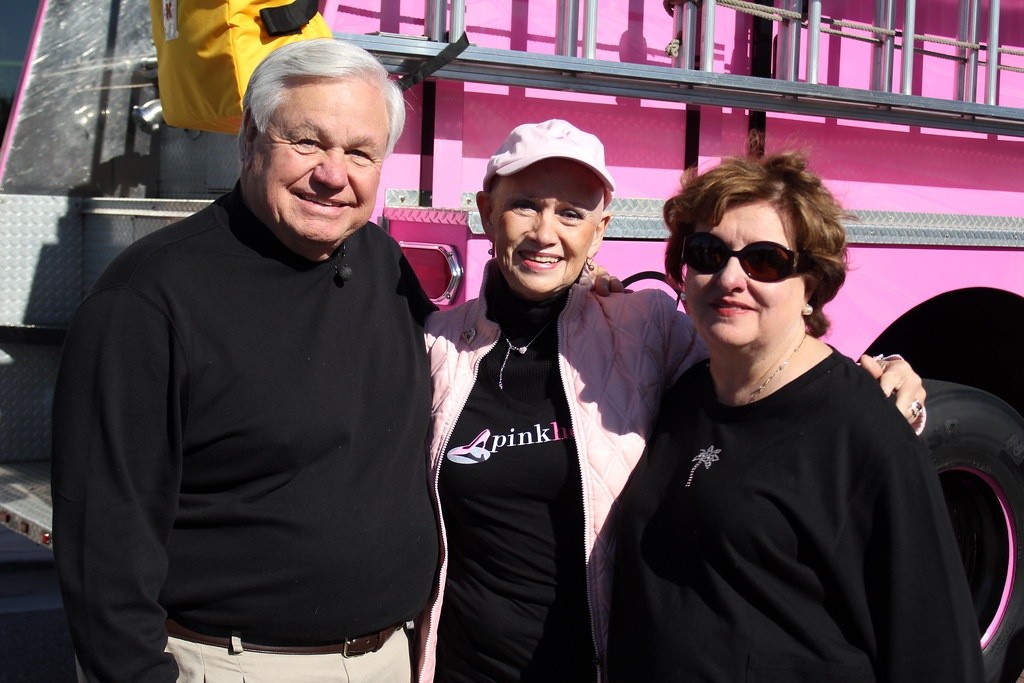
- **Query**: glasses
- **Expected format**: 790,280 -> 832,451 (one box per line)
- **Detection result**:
683,231 -> 814,283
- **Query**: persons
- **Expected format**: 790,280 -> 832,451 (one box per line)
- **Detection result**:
399,116 -> 935,681
612,150 -> 987,683
47,36 -> 634,682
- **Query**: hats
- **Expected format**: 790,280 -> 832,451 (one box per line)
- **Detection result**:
483,119 -> 616,211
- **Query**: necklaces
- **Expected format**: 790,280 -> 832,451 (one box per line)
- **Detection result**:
491,313 -> 554,392
741,334 -> 810,411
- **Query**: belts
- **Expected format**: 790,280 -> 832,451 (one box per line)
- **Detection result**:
164,616 -> 405,657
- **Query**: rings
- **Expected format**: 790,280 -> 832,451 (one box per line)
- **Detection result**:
910,398 -> 924,419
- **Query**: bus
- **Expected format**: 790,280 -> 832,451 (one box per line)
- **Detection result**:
0,0 -> 1024,682
